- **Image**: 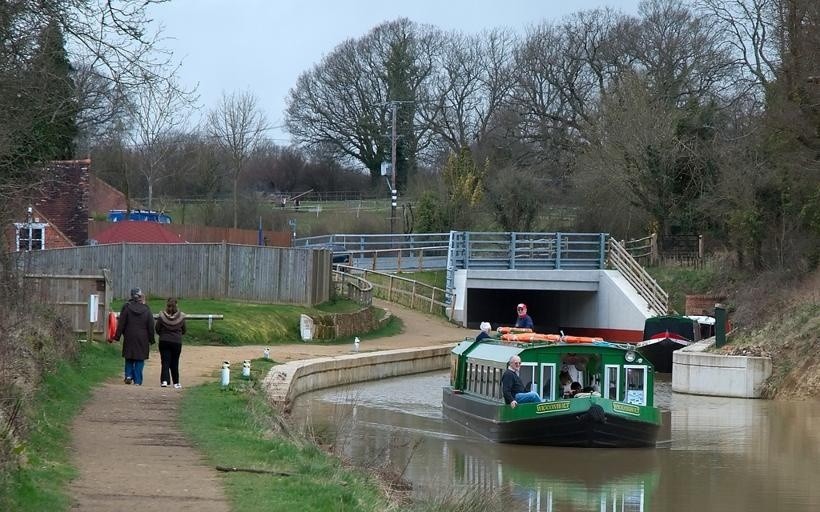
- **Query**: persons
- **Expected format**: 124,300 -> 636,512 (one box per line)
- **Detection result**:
155,297 -> 186,389
476,322 -> 492,341
502,355 -> 541,409
515,303 -> 534,328
113,287 -> 156,385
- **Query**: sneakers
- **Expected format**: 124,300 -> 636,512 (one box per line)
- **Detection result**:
125,377 -> 141,386
161,381 -> 168,387
173,382 -> 182,389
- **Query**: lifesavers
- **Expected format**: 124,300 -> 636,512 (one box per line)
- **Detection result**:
498,326 -> 603,343
107,311 -> 118,344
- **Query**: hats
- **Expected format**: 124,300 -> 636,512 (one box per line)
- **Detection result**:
517,303 -> 527,310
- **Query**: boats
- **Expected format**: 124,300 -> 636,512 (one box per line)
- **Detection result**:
636,314 -> 716,373
441,327 -> 660,447
